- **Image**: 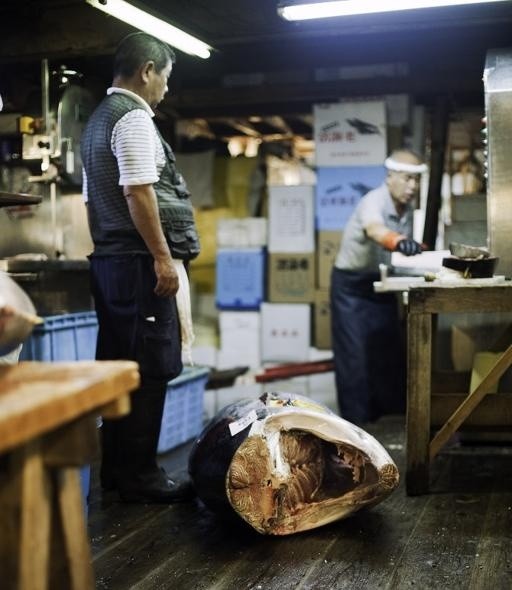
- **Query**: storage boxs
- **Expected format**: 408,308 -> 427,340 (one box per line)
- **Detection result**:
196,93 -> 402,368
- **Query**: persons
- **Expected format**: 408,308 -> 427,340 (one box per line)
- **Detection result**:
80,30 -> 203,505
327,145 -> 431,425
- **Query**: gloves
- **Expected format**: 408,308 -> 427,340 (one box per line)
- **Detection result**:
380,230 -> 428,256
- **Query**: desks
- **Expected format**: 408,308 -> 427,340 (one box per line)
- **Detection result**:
386,283 -> 511,497
0,358 -> 143,590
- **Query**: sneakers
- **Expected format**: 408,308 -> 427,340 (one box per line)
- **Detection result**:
100,467 -> 196,504
